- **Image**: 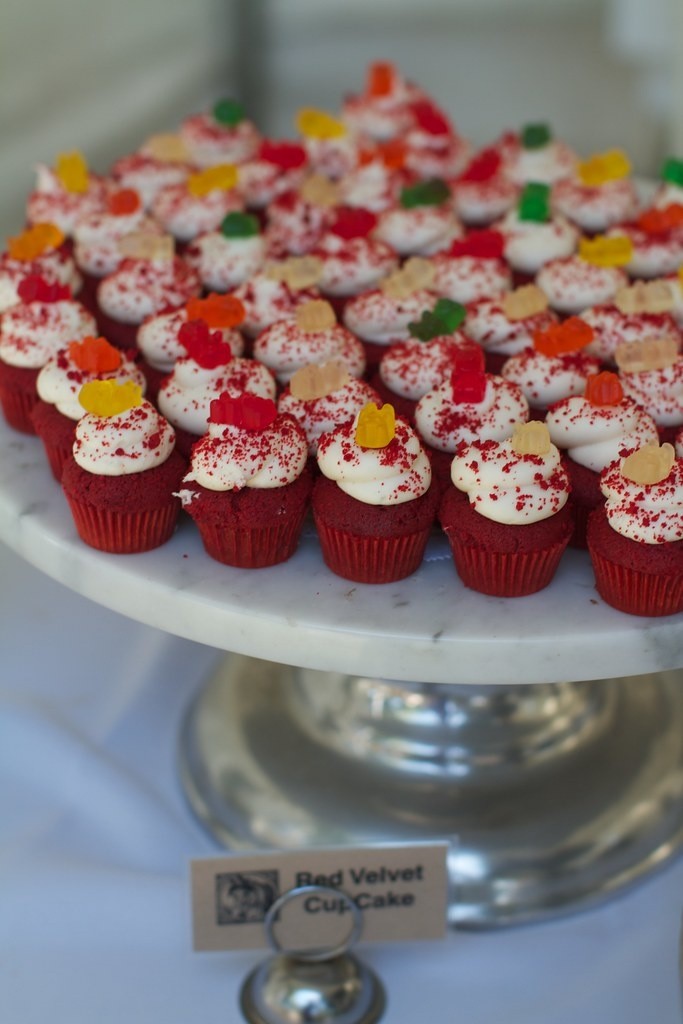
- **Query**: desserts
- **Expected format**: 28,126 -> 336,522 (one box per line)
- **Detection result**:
2,68 -> 682,617
